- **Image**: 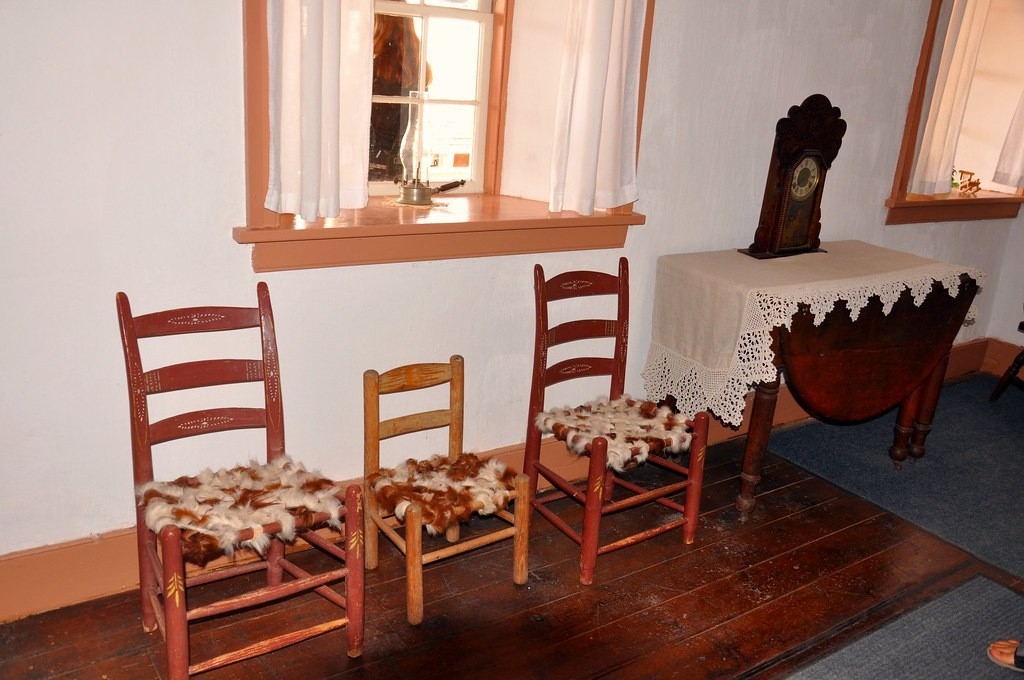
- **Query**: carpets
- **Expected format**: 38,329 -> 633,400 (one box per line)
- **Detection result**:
783,574 -> 1024,679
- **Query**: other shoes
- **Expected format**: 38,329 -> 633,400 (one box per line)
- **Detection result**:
988,638 -> 1024,673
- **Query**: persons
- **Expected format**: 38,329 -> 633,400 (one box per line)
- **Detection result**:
367,1 -> 435,181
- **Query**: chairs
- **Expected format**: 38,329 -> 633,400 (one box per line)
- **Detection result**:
519,259 -> 710,585
357,354 -> 531,626
988,321 -> 1024,403
114,279 -> 367,680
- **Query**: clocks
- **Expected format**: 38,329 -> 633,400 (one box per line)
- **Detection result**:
739,93 -> 847,261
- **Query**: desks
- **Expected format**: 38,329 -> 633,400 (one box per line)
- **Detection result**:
643,240 -> 984,527
